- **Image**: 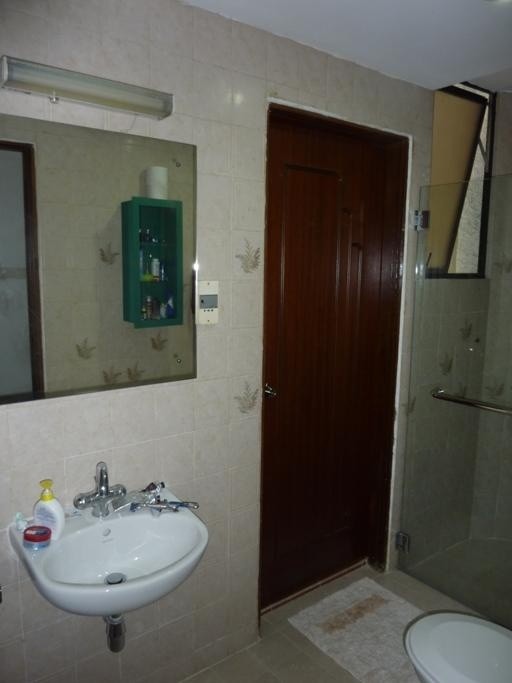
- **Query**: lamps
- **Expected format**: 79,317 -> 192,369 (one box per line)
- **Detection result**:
0,55 -> 176,121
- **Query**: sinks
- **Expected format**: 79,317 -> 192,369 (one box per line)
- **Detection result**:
8,488 -> 211,617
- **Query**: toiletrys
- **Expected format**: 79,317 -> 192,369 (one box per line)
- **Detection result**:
31,479 -> 65,542
138,227 -> 178,320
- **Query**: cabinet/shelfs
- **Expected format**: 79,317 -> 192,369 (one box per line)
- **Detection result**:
118,194 -> 183,328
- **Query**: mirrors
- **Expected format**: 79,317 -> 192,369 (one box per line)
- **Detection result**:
0,113 -> 197,404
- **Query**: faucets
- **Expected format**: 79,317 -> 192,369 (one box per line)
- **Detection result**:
74,462 -> 127,517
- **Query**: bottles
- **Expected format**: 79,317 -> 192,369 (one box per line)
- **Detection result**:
150,258 -> 160,277
141,295 -> 153,322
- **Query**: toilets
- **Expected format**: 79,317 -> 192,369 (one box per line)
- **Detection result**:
404,611 -> 512,683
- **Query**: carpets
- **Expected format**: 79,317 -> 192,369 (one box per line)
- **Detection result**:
285,575 -> 425,683
